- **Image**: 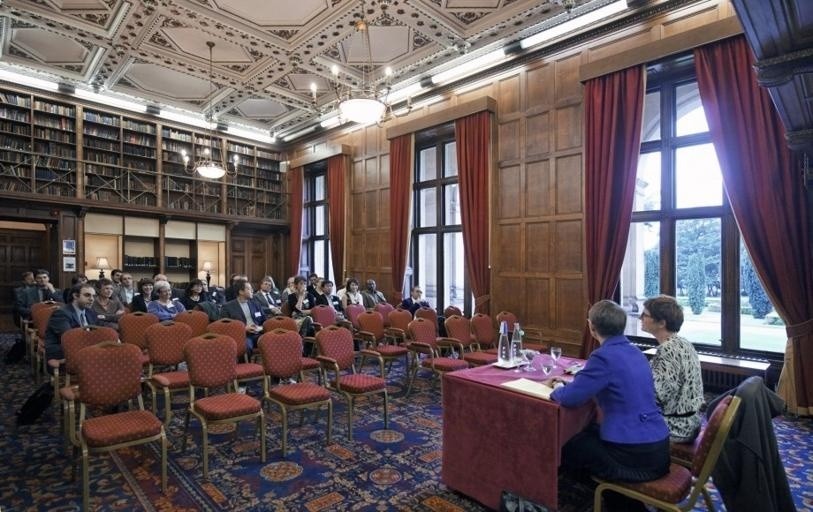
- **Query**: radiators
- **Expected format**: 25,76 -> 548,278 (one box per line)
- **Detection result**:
641,348 -> 771,392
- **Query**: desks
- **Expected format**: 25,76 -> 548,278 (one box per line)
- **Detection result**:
439,351 -> 597,512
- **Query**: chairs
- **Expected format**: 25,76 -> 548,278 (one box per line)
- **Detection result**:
665,375 -> 768,512
588,388 -> 745,512
69,341 -> 170,510
20,277 -> 525,450
313,325 -> 391,441
181,332 -> 270,480
256,325 -> 334,457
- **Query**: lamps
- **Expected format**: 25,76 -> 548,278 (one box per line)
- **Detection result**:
178,40 -> 239,182
197,260 -> 218,287
91,256 -> 113,278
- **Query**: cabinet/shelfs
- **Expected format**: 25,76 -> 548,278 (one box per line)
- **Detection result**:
0,80 -> 287,225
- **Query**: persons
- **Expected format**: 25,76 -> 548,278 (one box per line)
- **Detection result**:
15,270 -> 188,377
182,272 -> 386,394
638,294 -> 706,445
548,299 -> 672,511
400,286 -> 430,321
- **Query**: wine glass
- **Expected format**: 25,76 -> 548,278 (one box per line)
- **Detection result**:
549,346 -> 562,368
541,358 -> 554,383
524,350 -> 537,372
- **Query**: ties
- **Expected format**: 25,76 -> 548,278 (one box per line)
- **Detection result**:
264,292 -> 274,306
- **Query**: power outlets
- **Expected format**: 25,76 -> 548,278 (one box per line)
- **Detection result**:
304,0 -> 415,134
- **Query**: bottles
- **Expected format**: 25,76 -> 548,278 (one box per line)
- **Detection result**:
509,322 -> 522,365
512,351 -> 523,373
498,321 -> 511,364
